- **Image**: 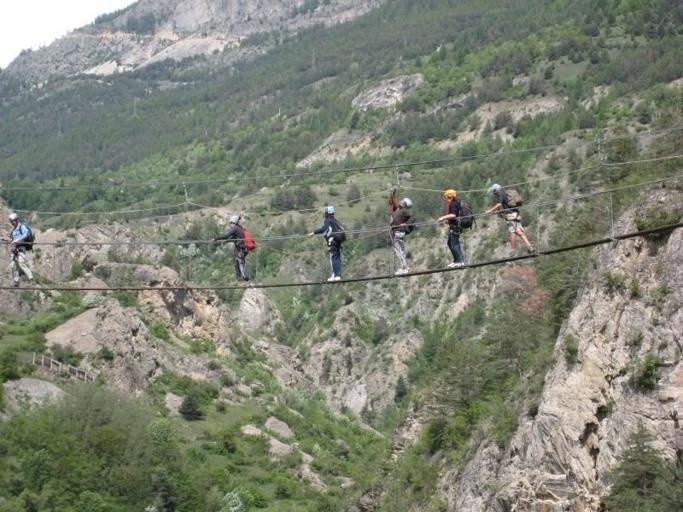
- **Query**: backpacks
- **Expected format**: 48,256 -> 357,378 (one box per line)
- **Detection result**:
19,223 -> 32,250
238,226 -> 255,249
453,200 -> 473,228
504,190 -> 522,207
402,212 -> 415,234
326,220 -> 345,242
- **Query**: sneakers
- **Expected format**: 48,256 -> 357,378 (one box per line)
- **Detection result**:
504,250 -> 516,258
394,268 -> 409,275
10,281 -> 17,287
328,274 -> 340,281
527,248 -> 535,254
447,261 -> 464,268
233,280 -> 255,287
26,280 -> 34,286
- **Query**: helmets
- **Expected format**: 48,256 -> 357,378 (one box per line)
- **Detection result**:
405,198 -> 412,208
230,216 -> 238,223
324,206 -> 333,213
493,184 -> 500,193
445,190 -> 456,197
8,213 -> 17,221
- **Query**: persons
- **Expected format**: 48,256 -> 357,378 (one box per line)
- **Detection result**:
0,213 -> 36,287
483,182 -> 533,258
212,215 -> 250,283
388,194 -> 416,273
439,189 -> 466,269
308,206 -> 346,282
